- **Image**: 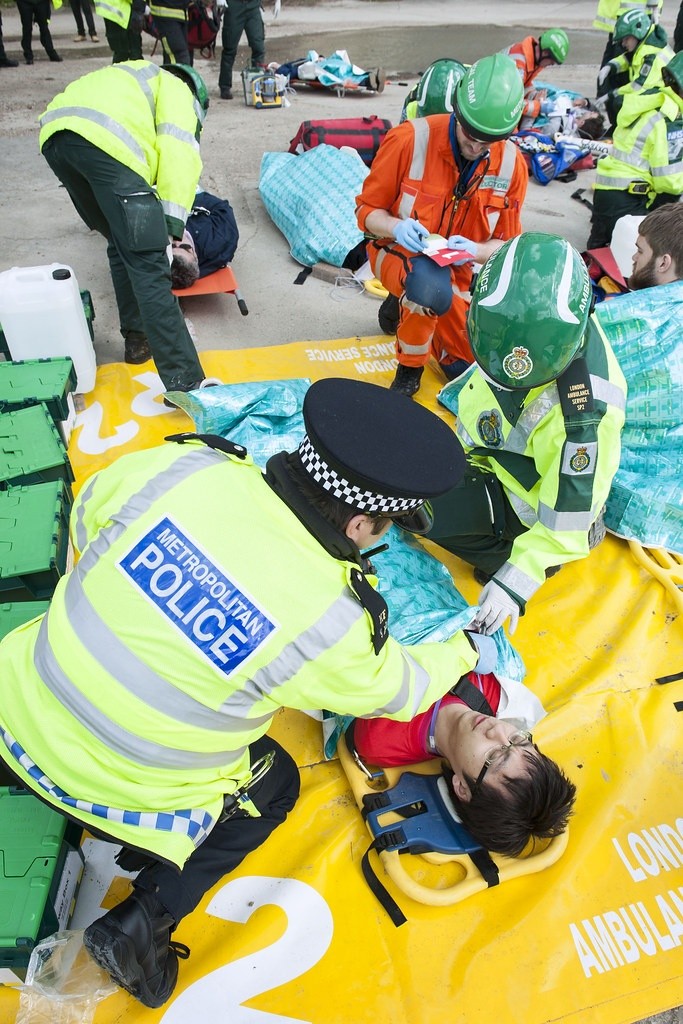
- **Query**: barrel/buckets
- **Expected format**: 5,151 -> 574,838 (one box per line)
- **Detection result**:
0,262 -> 98,395
609,213 -> 646,282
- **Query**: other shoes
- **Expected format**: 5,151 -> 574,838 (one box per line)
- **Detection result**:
377,66 -> 385,93
164,377 -> 218,408
92,35 -> 99,42
27,59 -> 33,64
221,88 -> 233,99
378,292 -> 400,335
389,363 -> 424,398
120,329 -> 151,364
368,73 -> 377,89
0,60 -> 18,67
73,35 -> 87,41
50,55 -> 63,61
474,564 -> 562,586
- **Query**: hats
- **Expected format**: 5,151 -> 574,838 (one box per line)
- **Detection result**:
298,377 -> 466,535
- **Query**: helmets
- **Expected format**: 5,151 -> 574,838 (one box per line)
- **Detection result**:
159,63 -> 210,117
453,54 -> 525,144
611,8 -> 651,41
466,230 -> 593,391
539,28 -> 569,65
417,58 -> 468,117
661,50 -> 683,95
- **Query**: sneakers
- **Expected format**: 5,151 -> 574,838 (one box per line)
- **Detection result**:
84,889 -> 190,1008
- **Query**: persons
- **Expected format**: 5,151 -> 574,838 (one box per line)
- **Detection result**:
0,10 -> 18,67
146,0 -> 194,66
456,231 -> 626,638
584,50 -> 683,249
591,203 -> 683,551
164,185 -> 238,289
594,0 -> 663,97
69,0 -> 98,43
520,88 -> 603,140
38,60 -> 209,405
328,533 -> 573,857
265,55 -> 384,93
16,0 -> 63,65
0,377 -> 497,1008
354,55 -> 526,400
215,0 -> 280,100
594,10 -> 675,128
501,30 -> 569,119
399,58 -> 466,123
93,0 -> 145,63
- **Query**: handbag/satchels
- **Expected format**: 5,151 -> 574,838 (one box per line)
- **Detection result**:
288,114 -> 393,169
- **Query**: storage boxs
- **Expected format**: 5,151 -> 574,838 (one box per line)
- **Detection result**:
0,290 -> 96,969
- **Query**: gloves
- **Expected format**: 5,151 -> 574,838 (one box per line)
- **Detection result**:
274,0 -> 280,20
392,218 -> 429,254
594,89 -> 618,108
217,0 -> 228,12
598,65 -> 611,85
448,235 -> 477,266
476,580 -> 519,637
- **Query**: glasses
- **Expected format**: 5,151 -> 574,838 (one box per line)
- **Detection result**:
172,243 -> 194,254
472,730 -> 533,796
